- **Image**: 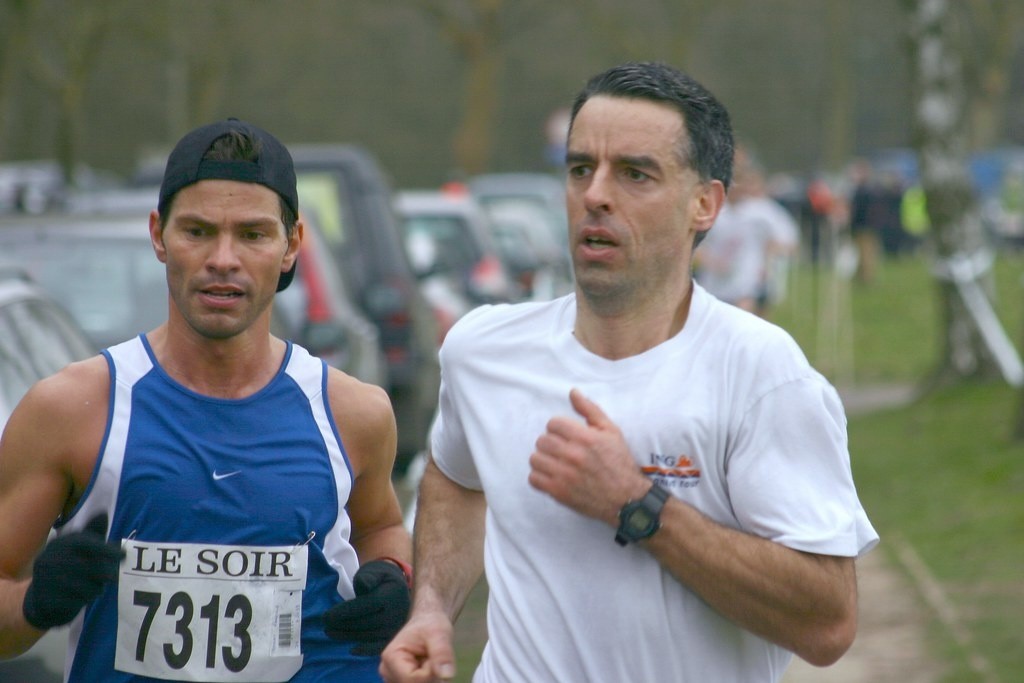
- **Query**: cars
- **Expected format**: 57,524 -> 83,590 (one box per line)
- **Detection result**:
0,132 -> 575,482
0,252 -> 102,442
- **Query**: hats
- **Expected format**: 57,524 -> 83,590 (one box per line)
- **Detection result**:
157,117 -> 299,293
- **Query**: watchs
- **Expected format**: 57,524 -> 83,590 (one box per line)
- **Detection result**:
614,483 -> 671,548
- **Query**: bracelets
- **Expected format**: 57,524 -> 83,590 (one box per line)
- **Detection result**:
372,556 -> 413,592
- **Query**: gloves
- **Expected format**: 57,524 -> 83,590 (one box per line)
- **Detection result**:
21,533 -> 126,629
319,558 -> 412,655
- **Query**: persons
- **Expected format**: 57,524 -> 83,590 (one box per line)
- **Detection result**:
377,61 -> 880,683
808,160 -> 930,286
689,171 -> 800,324
1,117 -> 414,682
